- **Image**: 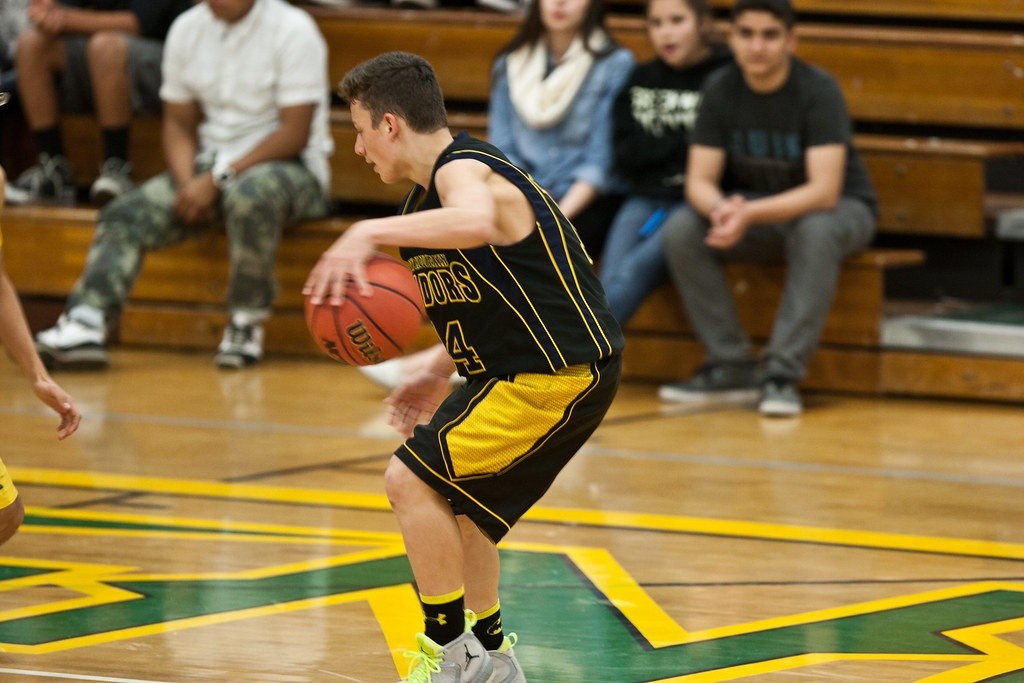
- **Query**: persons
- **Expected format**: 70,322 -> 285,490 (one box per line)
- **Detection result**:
486,0 -> 881,417
0,166 -> 81,546
0,0 -> 337,368
300,51 -> 625,683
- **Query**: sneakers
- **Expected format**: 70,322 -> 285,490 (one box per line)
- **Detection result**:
398,609 -> 496,683
659,367 -> 760,404
33,313 -> 109,366
487,632 -> 526,683
212,315 -> 265,368
758,381 -> 801,415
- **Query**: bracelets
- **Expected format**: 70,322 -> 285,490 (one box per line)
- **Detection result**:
211,165 -> 236,188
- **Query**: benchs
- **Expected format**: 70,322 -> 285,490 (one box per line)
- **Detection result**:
0,1 -> 1023,400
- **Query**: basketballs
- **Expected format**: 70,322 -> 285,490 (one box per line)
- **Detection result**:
303,250 -> 431,366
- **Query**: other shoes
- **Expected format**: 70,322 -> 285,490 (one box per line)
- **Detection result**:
90,163 -> 137,206
3,158 -> 77,207
